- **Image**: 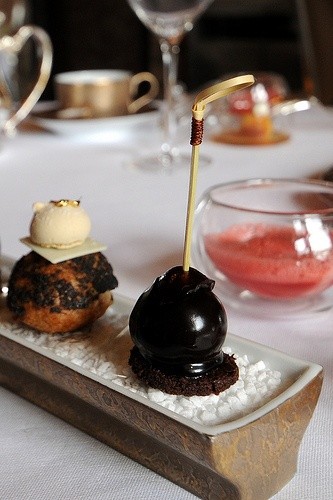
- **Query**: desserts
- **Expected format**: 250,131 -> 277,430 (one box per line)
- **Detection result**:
6,199 -> 118,335
127,265 -> 238,397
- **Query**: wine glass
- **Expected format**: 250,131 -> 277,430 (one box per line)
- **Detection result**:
126,0 -> 212,171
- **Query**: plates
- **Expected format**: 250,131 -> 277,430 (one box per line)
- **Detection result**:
0,251 -> 321,438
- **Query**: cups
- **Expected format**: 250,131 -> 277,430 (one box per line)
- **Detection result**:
211,71 -> 295,148
193,177 -> 333,317
55,69 -> 158,120
0,0 -> 53,139
